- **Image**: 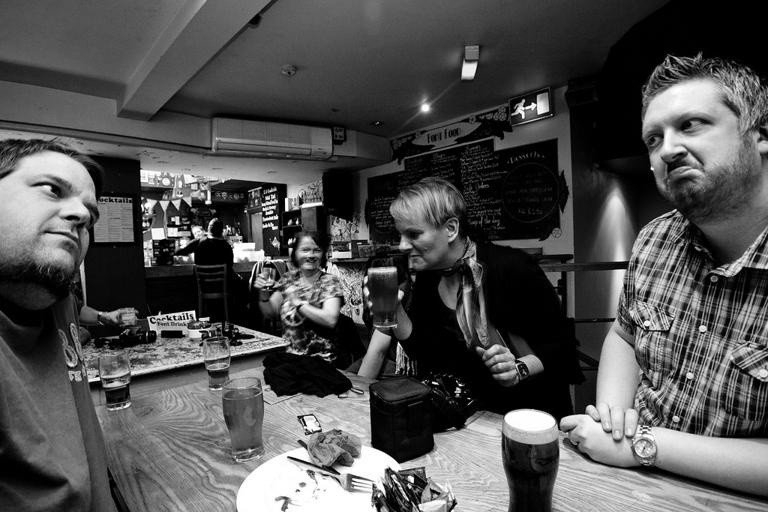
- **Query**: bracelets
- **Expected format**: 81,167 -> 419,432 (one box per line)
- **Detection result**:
96,311 -> 103,322
259,298 -> 269,303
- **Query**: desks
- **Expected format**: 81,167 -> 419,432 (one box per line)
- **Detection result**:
540,254 -> 574,317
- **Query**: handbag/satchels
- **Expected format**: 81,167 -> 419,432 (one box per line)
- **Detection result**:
419,372 -> 476,433
263,352 -> 352,398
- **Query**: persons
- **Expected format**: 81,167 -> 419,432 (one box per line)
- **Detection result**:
74,295 -> 140,329
173,217 -> 249,318
362,177 -> 586,431
1,138 -> 120,511
253,231 -> 346,366
558,54 -> 768,497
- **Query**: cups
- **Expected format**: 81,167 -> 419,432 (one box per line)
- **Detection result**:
119,308 -> 137,327
203,337 -> 232,390
98,349 -> 131,411
223,377 -> 265,463
368,266 -> 398,329
502,408 -> 559,512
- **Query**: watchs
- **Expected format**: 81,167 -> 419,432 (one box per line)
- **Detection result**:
296,300 -> 310,313
514,359 -> 530,381
631,424 -> 657,470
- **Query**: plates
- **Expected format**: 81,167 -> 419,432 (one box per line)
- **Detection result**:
236,445 -> 402,511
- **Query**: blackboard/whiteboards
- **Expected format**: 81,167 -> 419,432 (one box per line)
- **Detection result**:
262,185 -> 283,257
367,137 -> 560,248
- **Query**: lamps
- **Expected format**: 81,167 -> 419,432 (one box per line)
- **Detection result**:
459,44 -> 480,81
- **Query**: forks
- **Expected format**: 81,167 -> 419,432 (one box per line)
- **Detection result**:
286,454 -> 376,494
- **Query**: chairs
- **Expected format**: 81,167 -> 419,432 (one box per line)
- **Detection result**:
195,264 -> 228,320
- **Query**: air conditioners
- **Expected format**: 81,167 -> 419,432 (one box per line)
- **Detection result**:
211,117 -> 334,158
